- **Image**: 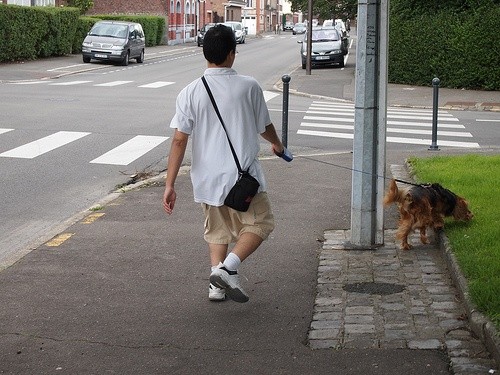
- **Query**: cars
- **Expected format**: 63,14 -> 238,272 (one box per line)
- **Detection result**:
226,21 -> 248,44
284,21 -> 294,31
296,28 -> 347,69
292,23 -> 307,35
196,22 -> 217,47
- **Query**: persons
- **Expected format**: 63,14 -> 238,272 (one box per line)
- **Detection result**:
163,24 -> 283,304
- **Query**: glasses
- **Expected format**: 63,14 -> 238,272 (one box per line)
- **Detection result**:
235,50 -> 239,56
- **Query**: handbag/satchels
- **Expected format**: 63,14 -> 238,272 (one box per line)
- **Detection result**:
224,170 -> 260,212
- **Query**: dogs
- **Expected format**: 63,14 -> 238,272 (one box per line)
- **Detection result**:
384,179 -> 474,253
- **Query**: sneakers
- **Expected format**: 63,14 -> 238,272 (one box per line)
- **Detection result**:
208,281 -> 228,301
209,261 -> 250,304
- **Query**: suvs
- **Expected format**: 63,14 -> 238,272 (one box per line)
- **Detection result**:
312,18 -> 319,26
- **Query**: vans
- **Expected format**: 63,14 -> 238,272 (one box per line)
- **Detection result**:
81,20 -> 146,66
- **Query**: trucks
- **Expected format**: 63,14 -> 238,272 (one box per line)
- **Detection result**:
323,19 -> 346,32
